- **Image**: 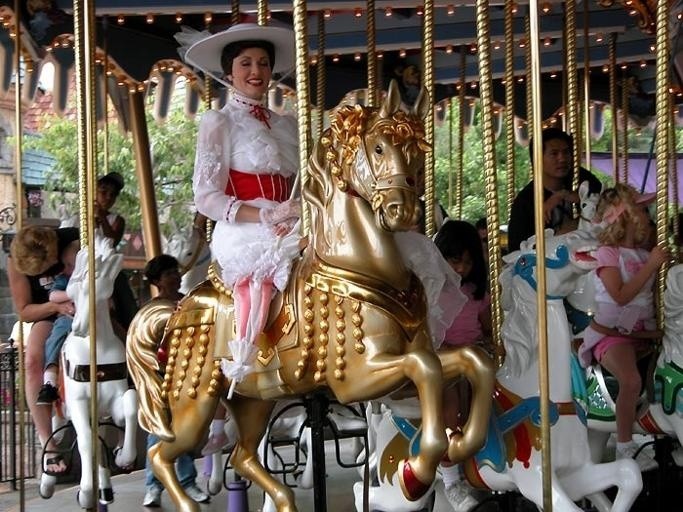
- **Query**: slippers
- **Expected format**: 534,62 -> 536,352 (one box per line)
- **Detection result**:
47,455 -> 69,476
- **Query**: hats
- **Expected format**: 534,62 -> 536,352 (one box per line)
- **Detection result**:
98,171 -> 124,189
593,190 -> 657,230
184,23 -> 295,73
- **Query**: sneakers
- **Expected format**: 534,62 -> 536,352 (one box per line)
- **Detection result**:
35,381 -> 59,405
201,433 -> 228,456
616,446 -> 658,471
444,480 -> 479,512
185,486 -> 210,502
143,489 -> 160,506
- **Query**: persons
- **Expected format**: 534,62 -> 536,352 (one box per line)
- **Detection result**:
33,233 -> 84,406
138,254 -> 213,505
429,219 -> 506,512
183,22 -> 315,284
577,184 -> 672,471
92,171 -> 125,246
474,217 -> 507,287
507,127 -> 603,259
6,224 -> 80,475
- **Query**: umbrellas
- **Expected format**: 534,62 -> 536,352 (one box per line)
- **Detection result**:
221,169 -> 305,399
